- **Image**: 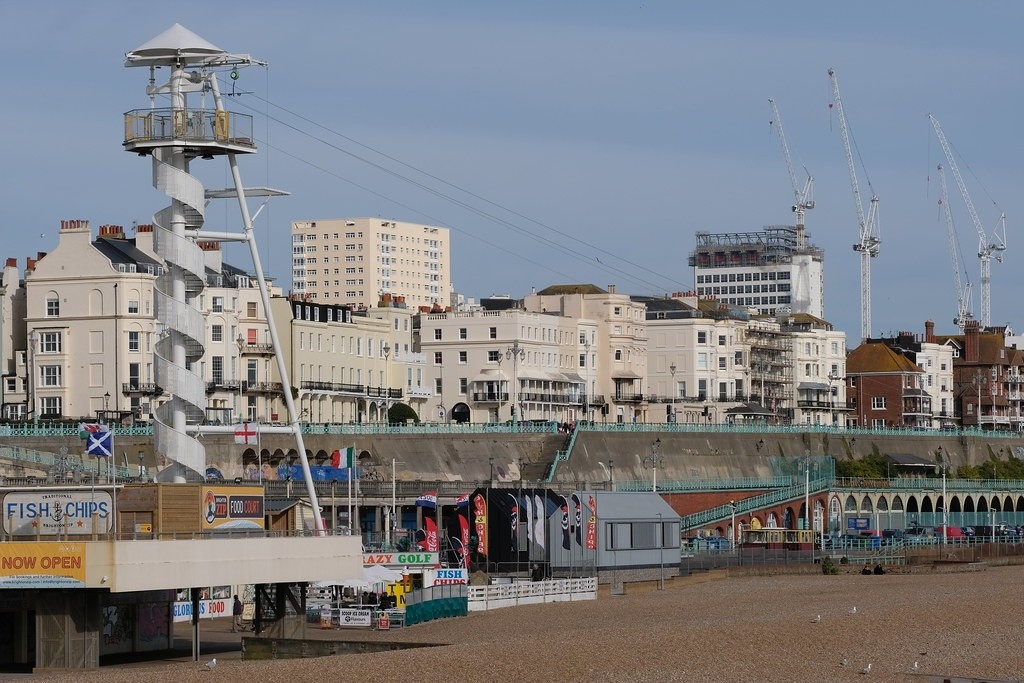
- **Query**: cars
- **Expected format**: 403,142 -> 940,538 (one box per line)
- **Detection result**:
816,523 -> 1024,548
702,537 -> 732,551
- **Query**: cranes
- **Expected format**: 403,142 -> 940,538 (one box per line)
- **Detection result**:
826,67 -> 885,344
767,97 -> 817,252
925,113 -> 1007,333
934,163 -> 976,335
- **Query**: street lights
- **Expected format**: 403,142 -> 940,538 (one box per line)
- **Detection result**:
286,454 -> 291,478
236,333 -> 245,423
506,339 -> 526,425
497,348 -> 504,413
643,438 -> 665,491
488,456 -> 524,480
382,345 -> 390,420
827,371 -> 834,426
744,367 -> 751,403
104,390 -> 111,419
670,362 -> 676,413
608,459 -> 614,482
138,450 -> 145,477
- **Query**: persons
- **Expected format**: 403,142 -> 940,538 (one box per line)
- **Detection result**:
363,591 -> 397,610
564,422 -> 568,433
229,595 -> 246,633
531,563 -> 543,581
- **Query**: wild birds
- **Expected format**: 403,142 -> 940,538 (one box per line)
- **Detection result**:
840,657 -> 847,667
206,658 -> 217,669
910,662 -> 919,673
848,607 -> 856,614
862,664 -> 872,674
811,615 -> 820,622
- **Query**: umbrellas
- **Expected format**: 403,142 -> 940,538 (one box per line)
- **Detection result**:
355,565 -> 402,592
316,579 -> 357,602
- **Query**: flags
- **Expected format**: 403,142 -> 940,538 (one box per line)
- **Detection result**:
585,495 -> 596,550
425,517 -> 439,552
535,495 -> 544,549
451,537 -> 464,566
507,492 -> 519,552
457,493 -> 468,508
524,495 -> 532,542
459,514 -> 468,554
78,423 -> 107,439
415,493 -> 437,509
234,423 -> 257,445
571,493 -> 582,545
559,494 -> 571,550
84,431 -> 112,456
474,494 -> 488,555
332,446 -> 354,468
